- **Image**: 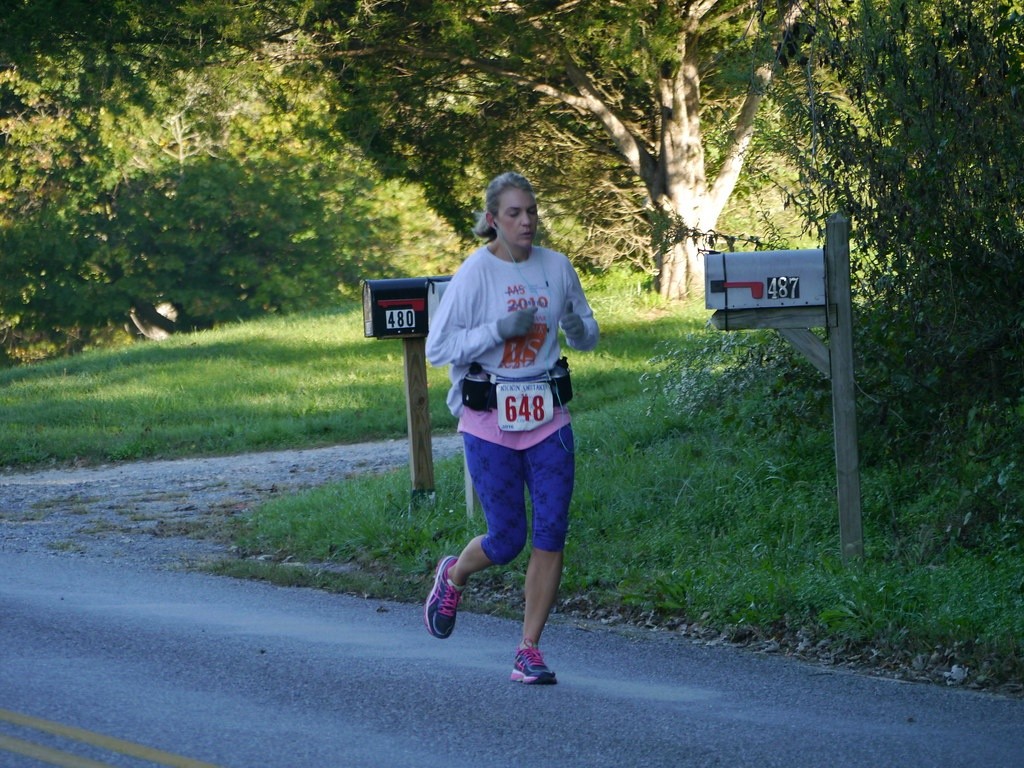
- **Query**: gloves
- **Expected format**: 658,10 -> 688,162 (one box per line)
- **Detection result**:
497,307 -> 538,340
561,301 -> 584,339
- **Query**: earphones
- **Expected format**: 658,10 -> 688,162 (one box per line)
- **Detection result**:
492,220 -> 498,228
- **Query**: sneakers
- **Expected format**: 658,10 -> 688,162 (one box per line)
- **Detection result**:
423,555 -> 467,638
510,638 -> 557,684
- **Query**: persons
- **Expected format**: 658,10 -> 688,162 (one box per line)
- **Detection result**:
424,173 -> 599,685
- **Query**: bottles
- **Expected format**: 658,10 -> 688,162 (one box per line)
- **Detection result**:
466,361 -> 489,381
552,356 -> 570,379
